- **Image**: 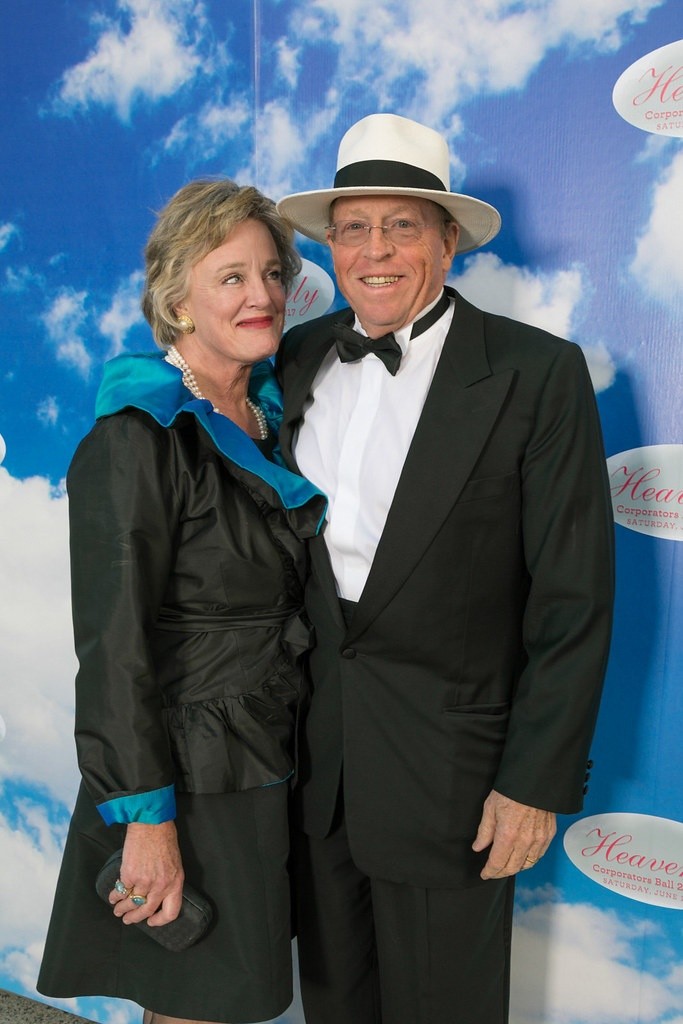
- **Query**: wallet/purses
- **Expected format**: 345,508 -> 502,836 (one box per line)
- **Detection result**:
95,847 -> 214,953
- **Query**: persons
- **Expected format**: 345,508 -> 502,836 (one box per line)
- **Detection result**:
35,176 -> 333,1024
275,114 -> 617,1024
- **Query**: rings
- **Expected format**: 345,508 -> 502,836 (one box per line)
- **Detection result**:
525,855 -> 537,864
115,880 -> 131,895
130,893 -> 147,905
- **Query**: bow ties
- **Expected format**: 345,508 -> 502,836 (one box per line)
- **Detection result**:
331,292 -> 451,377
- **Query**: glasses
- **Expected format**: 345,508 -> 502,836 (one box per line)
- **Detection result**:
324,219 -> 443,246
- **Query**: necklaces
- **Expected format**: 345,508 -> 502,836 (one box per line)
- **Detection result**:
162,345 -> 270,440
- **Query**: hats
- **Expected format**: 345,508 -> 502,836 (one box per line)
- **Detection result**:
275,113 -> 502,254
